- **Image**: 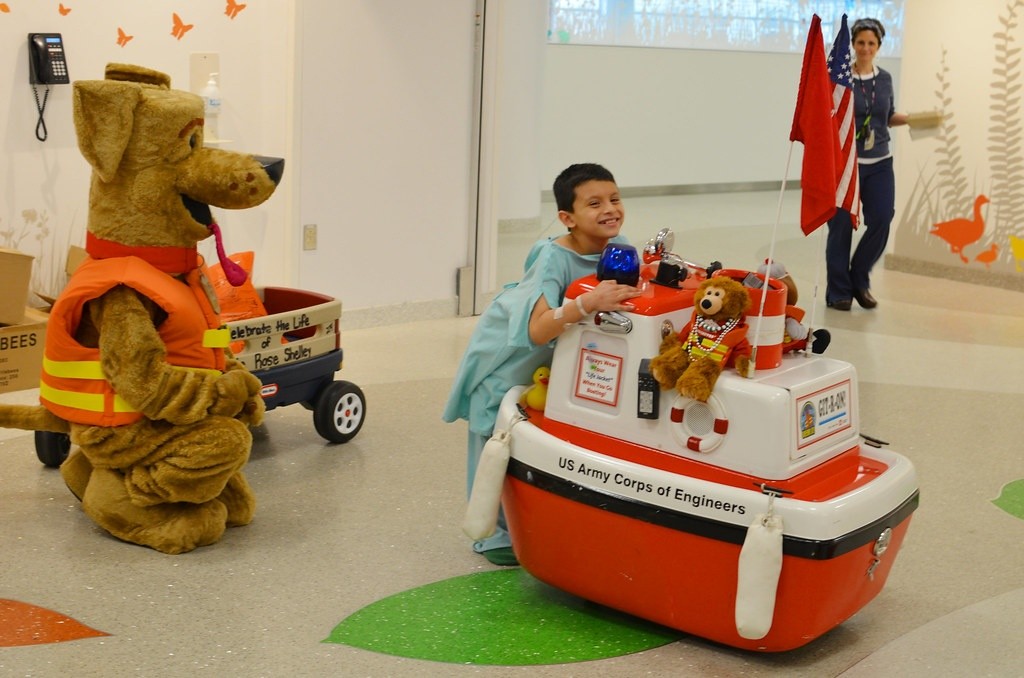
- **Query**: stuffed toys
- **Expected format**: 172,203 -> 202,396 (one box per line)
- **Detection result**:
757,258 -> 832,355
0,62 -> 286,554
649,277 -> 753,403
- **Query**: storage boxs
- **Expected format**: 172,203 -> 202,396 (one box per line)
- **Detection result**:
65,244 -> 90,285
0,309 -> 49,393
0,246 -> 36,324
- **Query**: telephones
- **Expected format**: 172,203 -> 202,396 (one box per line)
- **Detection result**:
28,33 -> 69,84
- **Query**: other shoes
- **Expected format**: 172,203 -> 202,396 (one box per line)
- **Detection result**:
483,545 -> 521,566
852,286 -> 876,308
832,297 -> 852,310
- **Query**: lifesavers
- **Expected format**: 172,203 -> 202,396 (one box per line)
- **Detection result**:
667,387 -> 732,454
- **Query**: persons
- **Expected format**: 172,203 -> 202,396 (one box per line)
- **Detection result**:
442,163 -> 641,566
826,18 -> 909,311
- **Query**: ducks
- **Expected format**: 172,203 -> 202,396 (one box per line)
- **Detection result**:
520,366 -> 550,411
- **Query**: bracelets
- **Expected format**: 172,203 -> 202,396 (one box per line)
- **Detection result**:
554,306 -> 573,330
576,294 -> 589,317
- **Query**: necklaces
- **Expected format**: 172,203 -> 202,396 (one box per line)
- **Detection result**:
688,315 -> 740,362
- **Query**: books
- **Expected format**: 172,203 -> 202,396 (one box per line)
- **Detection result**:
906,111 -> 944,141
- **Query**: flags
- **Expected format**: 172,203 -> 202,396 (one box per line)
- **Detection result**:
788,14 -> 844,236
827,12 -> 861,232
